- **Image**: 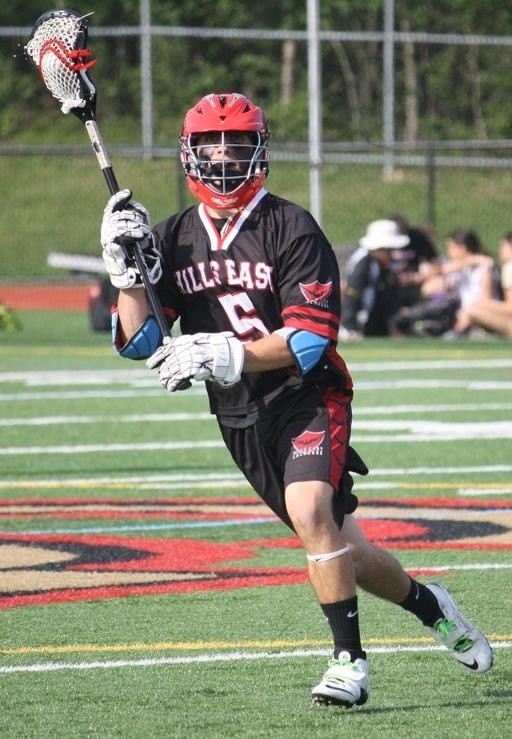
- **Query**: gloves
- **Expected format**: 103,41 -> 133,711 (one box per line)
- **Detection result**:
100,188 -> 163,289
146,330 -> 245,392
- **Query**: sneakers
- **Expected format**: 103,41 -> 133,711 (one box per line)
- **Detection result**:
312,647 -> 368,708
423,582 -> 493,673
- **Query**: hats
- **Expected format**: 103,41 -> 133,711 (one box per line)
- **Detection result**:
357,219 -> 411,250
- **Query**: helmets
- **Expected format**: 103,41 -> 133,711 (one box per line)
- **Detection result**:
179,92 -> 270,209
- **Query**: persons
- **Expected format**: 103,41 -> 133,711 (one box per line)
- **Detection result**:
98,92 -> 496,709
321,212 -> 512,342
